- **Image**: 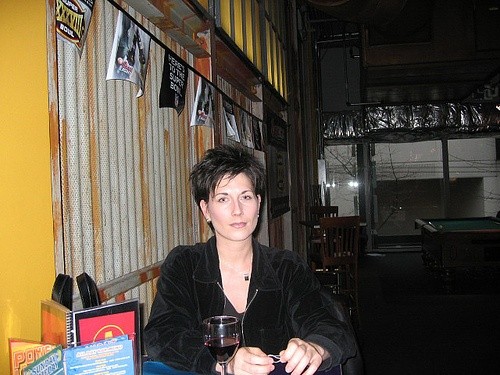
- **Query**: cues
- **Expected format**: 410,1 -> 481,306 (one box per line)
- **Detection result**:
429,222 -> 436,228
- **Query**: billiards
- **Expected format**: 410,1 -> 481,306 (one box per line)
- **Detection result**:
440,225 -> 443,229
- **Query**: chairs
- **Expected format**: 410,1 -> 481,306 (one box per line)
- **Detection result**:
319,287 -> 365,374
310,205 -> 363,340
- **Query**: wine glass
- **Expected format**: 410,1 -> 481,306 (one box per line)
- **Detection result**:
202,315 -> 239,375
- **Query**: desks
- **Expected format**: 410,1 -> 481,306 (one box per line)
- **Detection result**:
415,217 -> 500,293
300,221 -> 367,296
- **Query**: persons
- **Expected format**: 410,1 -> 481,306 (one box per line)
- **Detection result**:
144,143 -> 357,375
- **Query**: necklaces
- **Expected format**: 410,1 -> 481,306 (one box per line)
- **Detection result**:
219,250 -> 253,282
